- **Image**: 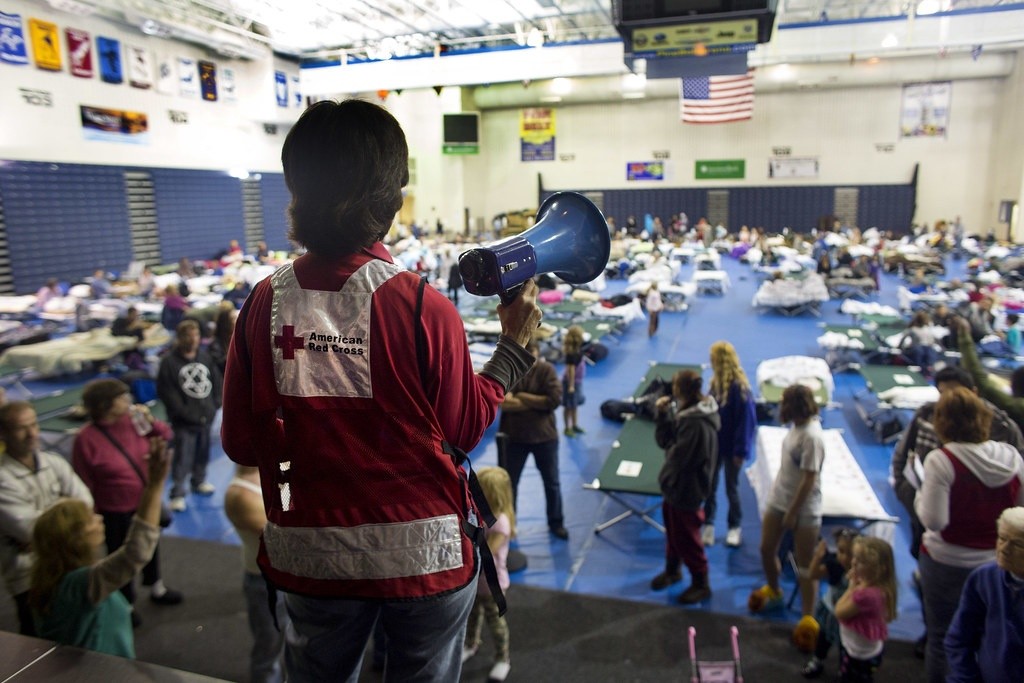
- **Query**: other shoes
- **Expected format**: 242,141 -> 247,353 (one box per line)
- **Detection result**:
700,525 -> 715,546
131,613 -> 143,629
462,644 -> 476,663
749,585 -> 782,612
191,481 -> 216,494
565,427 -> 575,437
548,526 -> 569,540
796,616 -> 819,648
650,571 -> 682,591
726,527 -> 741,546
804,657 -> 819,675
681,583 -> 710,605
913,570 -> 922,589
149,586 -> 185,607
573,425 -> 586,432
490,659 -> 511,681
169,496 -> 186,511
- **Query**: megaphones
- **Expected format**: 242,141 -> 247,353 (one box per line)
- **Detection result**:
460,191 -> 611,308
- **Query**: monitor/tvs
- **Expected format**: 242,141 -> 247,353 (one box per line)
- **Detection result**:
444,114 -> 480,144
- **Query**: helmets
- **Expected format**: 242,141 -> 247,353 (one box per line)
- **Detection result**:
80,378 -> 129,416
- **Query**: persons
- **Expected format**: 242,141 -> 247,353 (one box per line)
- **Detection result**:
383,210 -> 715,339
748,384 -> 826,650
891,312 -> 1024,683
493,337 -> 570,540
459,466 -> 517,683
70,377 -> 186,630
713,217 -> 1024,374
220,100 -> 545,683
0,402 -> 94,638
650,368 -> 722,606
156,320 -> 225,513
1,238 -> 308,370
30,432 -> 175,660
560,330 -> 586,436
801,524 -> 898,683
698,340 -> 758,547
224,462 -> 286,683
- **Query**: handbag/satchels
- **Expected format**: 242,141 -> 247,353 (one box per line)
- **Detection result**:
158,503 -> 172,528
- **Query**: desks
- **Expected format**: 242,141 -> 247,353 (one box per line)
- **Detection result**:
0,233 -> 1024,609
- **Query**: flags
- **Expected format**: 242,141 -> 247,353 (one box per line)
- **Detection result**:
681,67 -> 757,127
